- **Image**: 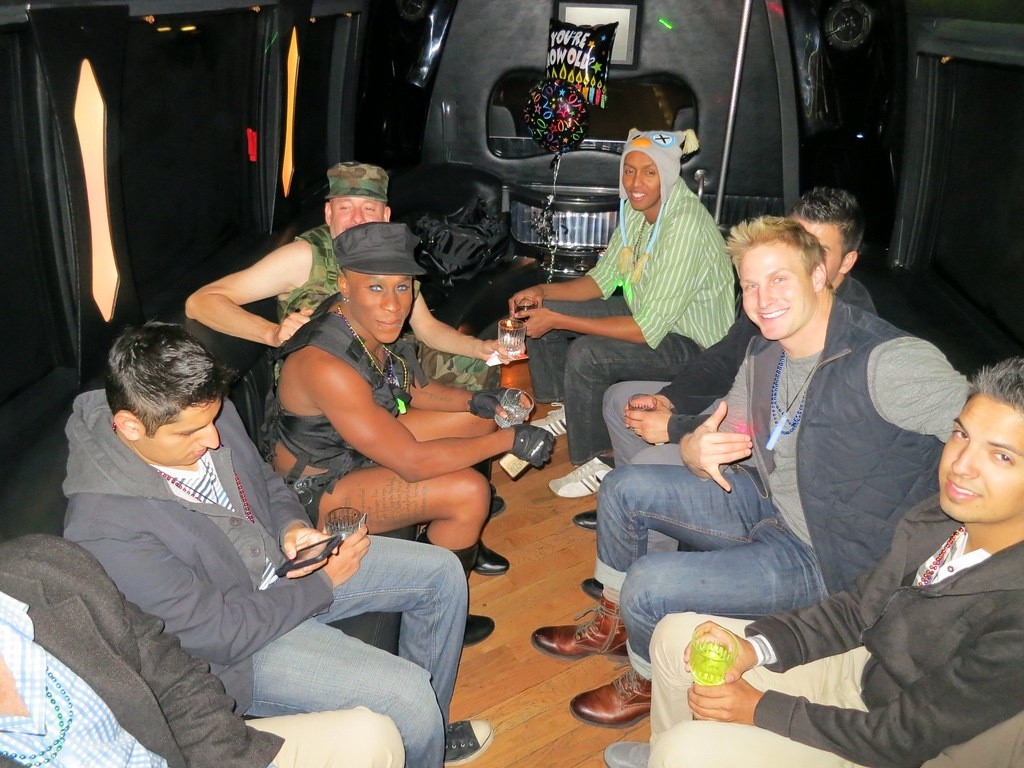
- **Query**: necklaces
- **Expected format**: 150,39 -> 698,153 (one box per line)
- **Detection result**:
113,423 -> 255,524
633,216 -> 656,265
917,526 -> 965,586
770,349 -> 820,437
336,307 -> 409,416
0,666 -> 73,768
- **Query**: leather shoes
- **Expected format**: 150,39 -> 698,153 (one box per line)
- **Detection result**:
583,578 -> 605,603
571,508 -> 597,532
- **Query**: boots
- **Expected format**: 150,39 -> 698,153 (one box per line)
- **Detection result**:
530,585 -> 629,663
469,456 -> 510,577
570,663 -> 652,729
422,527 -> 495,648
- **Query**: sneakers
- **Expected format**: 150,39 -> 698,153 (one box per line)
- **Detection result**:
444,718 -> 493,766
604,740 -> 649,768
528,401 -> 567,436
547,457 -> 614,497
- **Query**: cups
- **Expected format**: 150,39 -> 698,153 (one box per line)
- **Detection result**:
498,319 -> 527,357
689,625 -> 738,687
323,506 -> 363,542
494,387 -> 534,430
513,295 -> 538,323
628,394 -> 658,412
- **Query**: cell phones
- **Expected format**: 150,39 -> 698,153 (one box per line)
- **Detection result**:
276,534 -> 343,577
498,452 -> 529,480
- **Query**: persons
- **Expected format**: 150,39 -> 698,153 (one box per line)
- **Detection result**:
261,220 -> 557,650
63,320 -> 493,768
572,188 -> 878,603
185,160 -> 526,492
530,214 -> 973,728
507,128 -> 736,499
0,535 -> 406,768
604,357 -> 1024,768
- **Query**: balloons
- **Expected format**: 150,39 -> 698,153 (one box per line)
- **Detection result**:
545,18 -> 619,109
523,77 -> 589,156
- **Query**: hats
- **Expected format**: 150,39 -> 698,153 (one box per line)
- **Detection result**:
619,127 -> 699,284
324,162 -> 388,204
331,221 -> 427,276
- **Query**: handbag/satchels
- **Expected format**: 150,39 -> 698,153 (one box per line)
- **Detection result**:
420,208 -> 513,288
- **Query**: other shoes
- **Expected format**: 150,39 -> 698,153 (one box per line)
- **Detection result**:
491,483 -> 496,496
487,498 -> 505,518
